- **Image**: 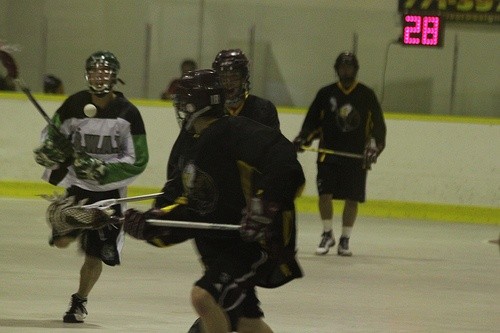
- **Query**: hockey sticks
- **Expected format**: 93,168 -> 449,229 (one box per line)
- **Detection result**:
301,145 -> 363,159
45,202 -> 240,248
38,192 -> 166,213
0,51 -> 76,156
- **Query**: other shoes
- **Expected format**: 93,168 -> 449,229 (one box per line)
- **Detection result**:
337,236 -> 352,256
63,293 -> 88,323
315,229 -> 336,255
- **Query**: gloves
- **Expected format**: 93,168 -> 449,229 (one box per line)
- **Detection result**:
71,148 -> 105,186
32,139 -> 67,169
123,208 -> 167,239
292,137 -> 310,153
240,197 -> 282,246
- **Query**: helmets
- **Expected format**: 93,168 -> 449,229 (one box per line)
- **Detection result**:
333,51 -> 359,84
181,59 -> 196,72
169,69 -> 214,129
212,48 -> 251,101
84,51 -> 120,97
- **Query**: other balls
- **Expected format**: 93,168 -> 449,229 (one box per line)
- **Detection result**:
84,104 -> 97,118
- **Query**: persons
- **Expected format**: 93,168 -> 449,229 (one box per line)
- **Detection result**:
43,74 -> 63,94
292,50 -> 387,256
0,50 -> 18,92
124,48 -> 306,333
33,51 -> 149,323
